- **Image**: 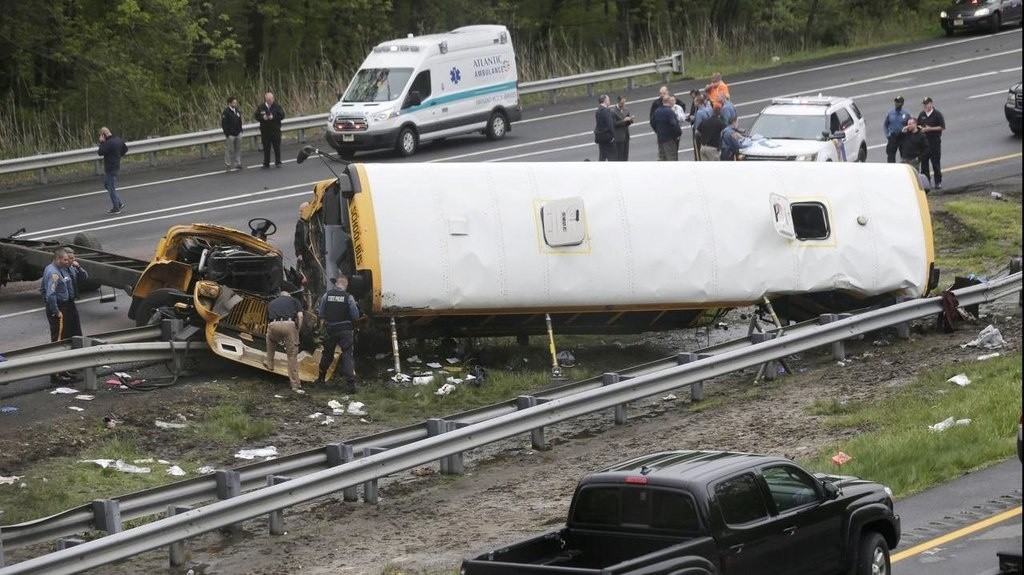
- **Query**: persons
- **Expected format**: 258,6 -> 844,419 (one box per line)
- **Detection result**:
649,72 -> 740,161
884,95 -> 911,162
613,95 -> 634,160
263,291 -> 303,390
98,127 -> 128,214
917,97 -> 944,188
40,249 -> 78,383
61,246 -> 88,336
595,94 -> 617,162
897,118 -> 928,171
254,92 -> 285,168
315,278 -> 358,394
221,96 -> 243,171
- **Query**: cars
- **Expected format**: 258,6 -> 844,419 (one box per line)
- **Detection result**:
939,0 -> 1023,38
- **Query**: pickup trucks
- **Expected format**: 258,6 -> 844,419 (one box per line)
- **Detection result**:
461,447 -> 903,574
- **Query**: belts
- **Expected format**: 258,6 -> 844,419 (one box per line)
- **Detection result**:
269,317 -> 295,322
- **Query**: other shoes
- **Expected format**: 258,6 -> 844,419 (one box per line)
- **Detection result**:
291,384 -> 301,390
235,165 -> 245,169
275,162 -> 282,168
262,163 -> 270,168
116,203 -> 125,209
934,180 -> 942,188
107,207 -> 121,215
226,165 -> 230,171
263,359 -> 275,371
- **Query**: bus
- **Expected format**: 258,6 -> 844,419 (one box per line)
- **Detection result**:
295,162 -> 937,345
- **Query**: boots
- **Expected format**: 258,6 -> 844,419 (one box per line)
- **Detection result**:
344,379 -> 358,394
315,371 -> 326,386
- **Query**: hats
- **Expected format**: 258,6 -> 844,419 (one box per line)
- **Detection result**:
921,96 -> 933,105
895,95 -> 904,102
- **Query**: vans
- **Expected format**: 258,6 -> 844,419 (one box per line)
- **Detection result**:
734,95 -> 867,161
326,23 -> 527,159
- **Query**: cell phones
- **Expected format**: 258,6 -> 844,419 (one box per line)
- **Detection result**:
103,133 -> 107,137
